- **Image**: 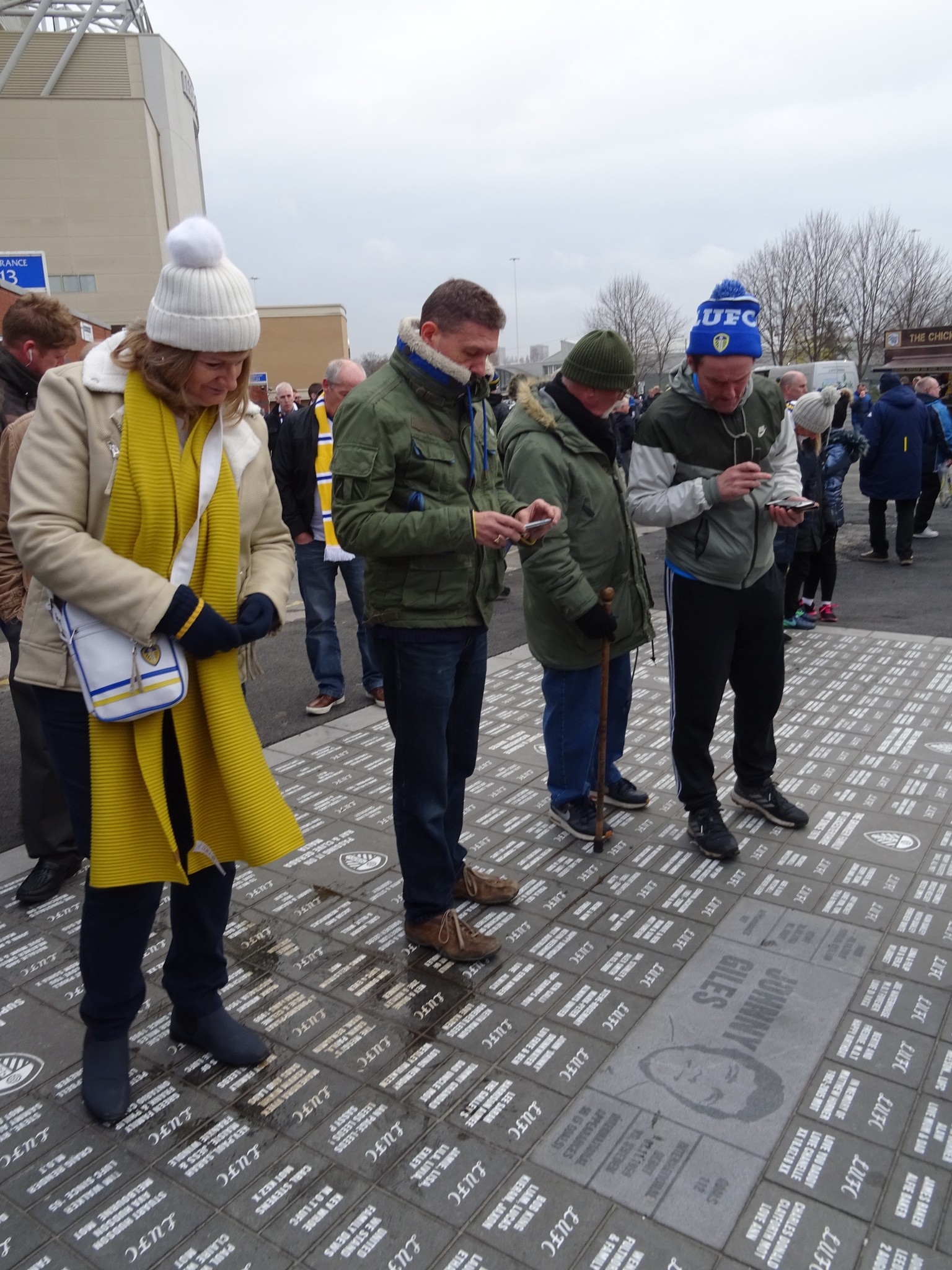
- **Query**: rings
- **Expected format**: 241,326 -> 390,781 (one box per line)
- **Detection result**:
494,534 -> 501,544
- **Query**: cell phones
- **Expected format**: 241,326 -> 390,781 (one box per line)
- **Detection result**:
764,499 -> 814,510
524,518 -> 552,530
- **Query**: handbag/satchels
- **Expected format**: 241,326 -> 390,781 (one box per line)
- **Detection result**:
937,462 -> 952,508
50,596 -> 187,722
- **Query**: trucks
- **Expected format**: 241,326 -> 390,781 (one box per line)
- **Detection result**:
751,360 -> 861,432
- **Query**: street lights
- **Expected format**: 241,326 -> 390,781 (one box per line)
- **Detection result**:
509,256 -> 521,364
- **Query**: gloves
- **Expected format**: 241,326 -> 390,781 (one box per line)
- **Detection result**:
158,582 -> 242,659
229,594 -> 277,645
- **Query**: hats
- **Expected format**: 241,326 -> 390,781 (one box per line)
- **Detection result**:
145,218 -> 262,352
560,329 -> 636,390
792,385 -> 843,435
831,388 -> 852,429
489,370 -> 499,385
879,372 -> 901,393
685,278 -> 763,358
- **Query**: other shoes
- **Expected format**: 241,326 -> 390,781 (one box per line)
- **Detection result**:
82,1028 -> 130,1124
858,550 -> 912,565
168,1008 -> 270,1065
501,586 -> 512,597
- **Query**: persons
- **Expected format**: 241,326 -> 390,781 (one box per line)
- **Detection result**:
497,329 -> 650,840
292,387 -> 302,405
849,383 -> 873,434
486,370 -> 511,597
330,278 -> 562,963
308,382 -> 323,405
778,369 -> 820,630
940,381 -> 952,507
486,368 -> 952,424
0,291 -> 80,426
912,376 -> 952,537
627,278 -> 821,860
263,381 -> 302,458
8,213 -> 299,1122
781,385 -> 842,644
0,406 -> 90,903
273,359 -> 387,716
797,385 -> 871,622
857,373 -> 931,565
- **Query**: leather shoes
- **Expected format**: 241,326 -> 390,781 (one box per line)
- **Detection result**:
17,858 -> 82,901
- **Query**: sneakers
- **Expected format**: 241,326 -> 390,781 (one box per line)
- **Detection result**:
306,688 -> 384,716
455,867 -> 520,905
686,807 -> 740,858
589,779 -> 650,809
782,599 -> 838,629
404,912 -> 501,961
730,777 -> 810,828
913,527 -> 939,538
549,797 -> 614,842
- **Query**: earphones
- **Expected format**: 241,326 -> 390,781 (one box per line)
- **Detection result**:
27,348 -> 32,362
